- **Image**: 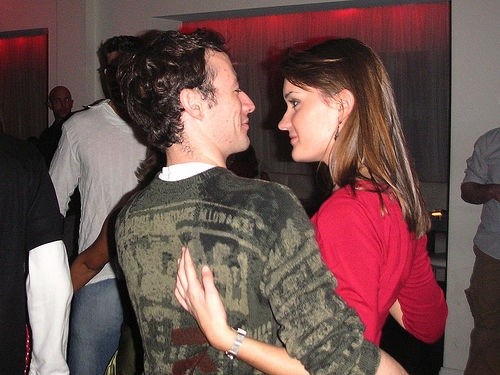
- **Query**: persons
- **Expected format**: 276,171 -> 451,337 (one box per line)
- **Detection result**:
174,36 -> 448,375
0,34 -> 271,375
114,25 -> 409,375
460,128 -> 500,375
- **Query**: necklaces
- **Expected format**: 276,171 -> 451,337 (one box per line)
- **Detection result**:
332,183 -> 337,188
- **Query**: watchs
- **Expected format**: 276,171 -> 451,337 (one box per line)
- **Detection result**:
225,328 -> 246,359
